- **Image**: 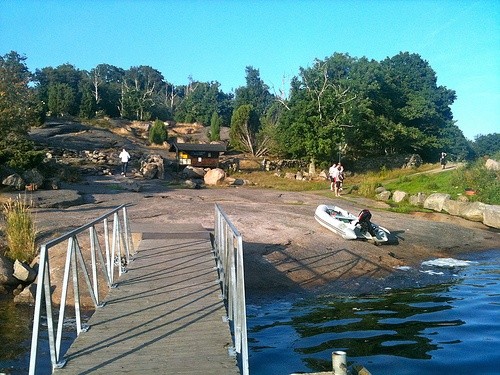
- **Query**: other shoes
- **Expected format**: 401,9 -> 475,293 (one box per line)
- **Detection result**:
121,172 -> 126,177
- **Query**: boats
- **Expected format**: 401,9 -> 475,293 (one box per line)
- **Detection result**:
314,204 -> 391,243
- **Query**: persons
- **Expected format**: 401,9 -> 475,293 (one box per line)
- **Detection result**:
335,168 -> 343,197
338,163 -> 344,191
328,163 -> 336,191
442,154 -> 447,170
119,149 -> 130,176
333,164 -> 340,183
